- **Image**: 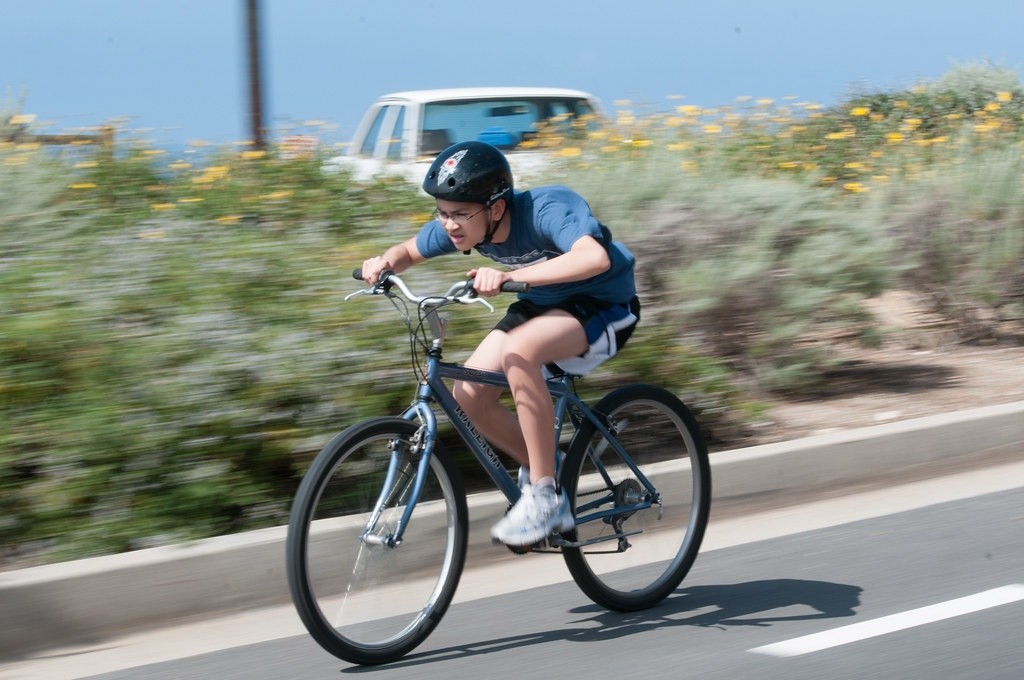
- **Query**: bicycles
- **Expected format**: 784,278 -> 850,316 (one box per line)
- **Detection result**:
285,267 -> 713,665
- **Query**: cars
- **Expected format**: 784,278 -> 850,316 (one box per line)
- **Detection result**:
324,87 -> 617,201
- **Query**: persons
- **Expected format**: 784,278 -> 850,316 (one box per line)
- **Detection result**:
361,140 -> 641,545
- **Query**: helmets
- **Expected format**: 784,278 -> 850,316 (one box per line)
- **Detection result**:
422,141 -> 514,207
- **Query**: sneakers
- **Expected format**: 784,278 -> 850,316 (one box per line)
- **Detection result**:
490,476 -> 576,548
516,451 -> 567,492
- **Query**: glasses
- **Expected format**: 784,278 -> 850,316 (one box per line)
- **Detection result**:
432,208 -> 491,225
